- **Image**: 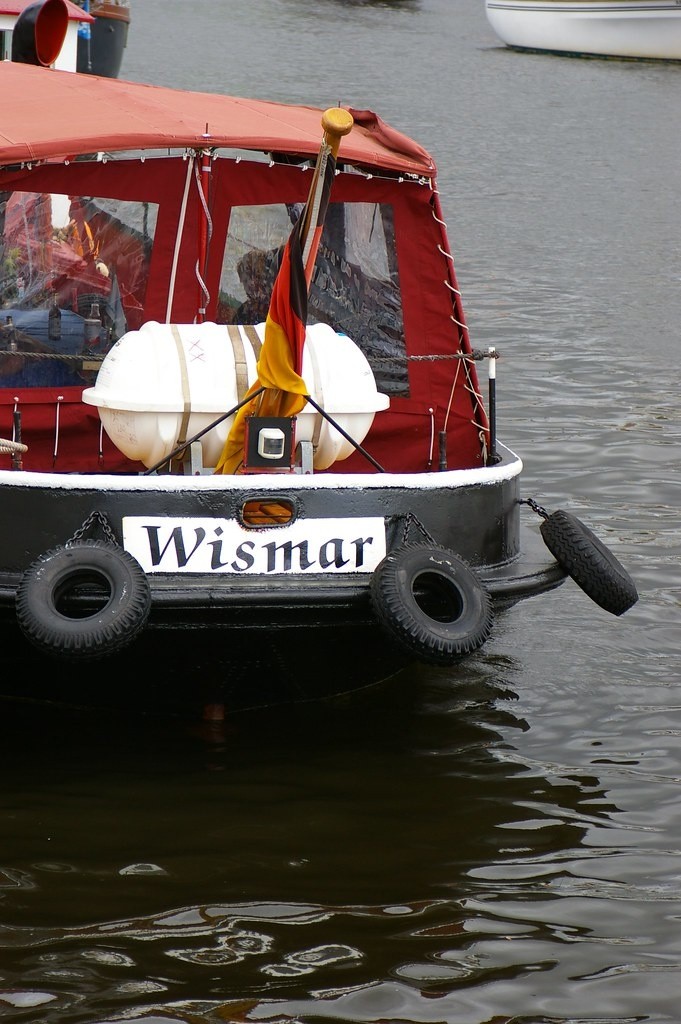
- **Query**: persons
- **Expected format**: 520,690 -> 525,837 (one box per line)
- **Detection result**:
231,248 -> 282,324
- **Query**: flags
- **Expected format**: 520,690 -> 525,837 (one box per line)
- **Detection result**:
216,128 -> 332,476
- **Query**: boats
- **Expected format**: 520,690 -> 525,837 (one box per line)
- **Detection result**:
0,54 -> 645,672
